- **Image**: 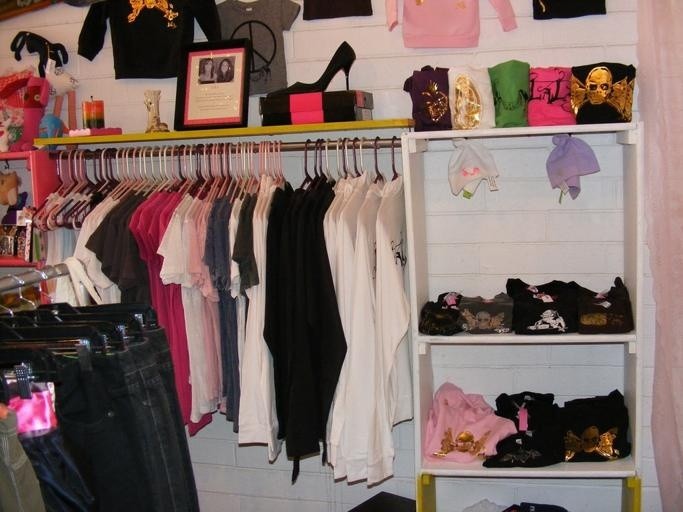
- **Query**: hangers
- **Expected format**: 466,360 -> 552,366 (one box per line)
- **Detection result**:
32,136 -> 398,232
0,265 -> 159,405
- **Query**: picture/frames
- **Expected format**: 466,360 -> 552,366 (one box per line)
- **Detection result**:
174,38 -> 251,130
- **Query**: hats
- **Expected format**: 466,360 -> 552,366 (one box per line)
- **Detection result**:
546,134 -> 600,200
448,137 -> 499,199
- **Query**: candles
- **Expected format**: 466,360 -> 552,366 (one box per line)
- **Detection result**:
82,96 -> 104,128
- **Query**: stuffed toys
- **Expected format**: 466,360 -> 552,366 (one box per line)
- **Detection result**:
0,171 -> 21,208
0,29 -> 79,156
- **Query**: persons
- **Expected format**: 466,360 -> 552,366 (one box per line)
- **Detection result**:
215,59 -> 234,84
198,58 -> 217,84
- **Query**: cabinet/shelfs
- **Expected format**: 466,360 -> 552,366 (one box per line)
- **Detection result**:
0,150 -> 62,305
401,122 -> 643,512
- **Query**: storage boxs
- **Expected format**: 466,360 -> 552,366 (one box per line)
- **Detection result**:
264,90 -> 374,125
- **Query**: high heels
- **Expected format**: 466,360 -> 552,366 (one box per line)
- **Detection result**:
267,42 -> 356,96
